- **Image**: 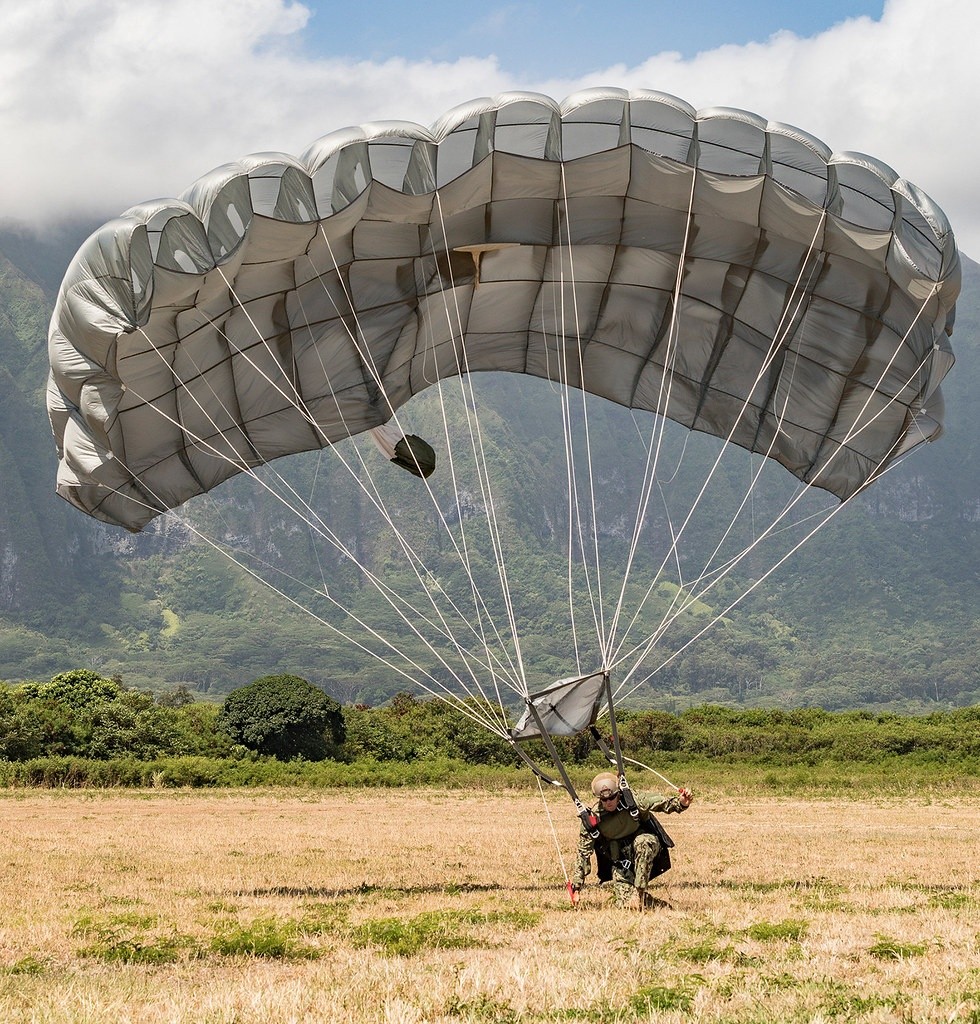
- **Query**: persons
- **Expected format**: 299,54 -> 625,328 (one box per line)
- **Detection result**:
568,772 -> 695,909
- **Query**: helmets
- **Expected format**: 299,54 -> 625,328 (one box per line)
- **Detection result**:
591,773 -> 621,799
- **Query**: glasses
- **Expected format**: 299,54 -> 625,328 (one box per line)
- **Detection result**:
602,792 -> 619,802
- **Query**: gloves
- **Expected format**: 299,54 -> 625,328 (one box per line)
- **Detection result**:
571,883 -> 582,894
